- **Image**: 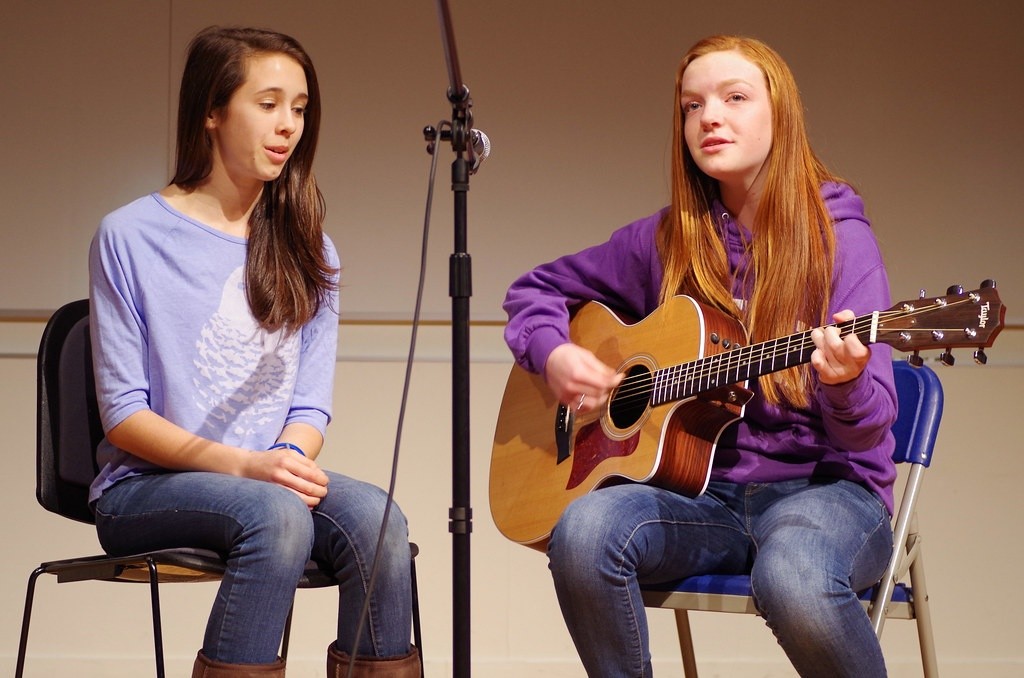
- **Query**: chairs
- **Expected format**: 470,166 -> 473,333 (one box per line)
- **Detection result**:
14,298 -> 424,678
639,359 -> 943,678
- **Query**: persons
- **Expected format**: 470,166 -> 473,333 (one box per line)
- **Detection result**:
86,24 -> 420,678
501,35 -> 898,678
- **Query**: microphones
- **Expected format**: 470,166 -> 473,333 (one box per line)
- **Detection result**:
443,129 -> 491,165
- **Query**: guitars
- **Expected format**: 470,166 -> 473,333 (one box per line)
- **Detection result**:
487,274 -> 1007,558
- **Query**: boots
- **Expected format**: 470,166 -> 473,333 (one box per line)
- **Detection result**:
326,639 -> 421,678
191,648 -> 286,678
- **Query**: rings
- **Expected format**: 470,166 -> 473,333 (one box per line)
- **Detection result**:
577,402 -> 584,410
579,393 -> 585,403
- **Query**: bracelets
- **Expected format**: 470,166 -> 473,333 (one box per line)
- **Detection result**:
268,442 -> 307,458
